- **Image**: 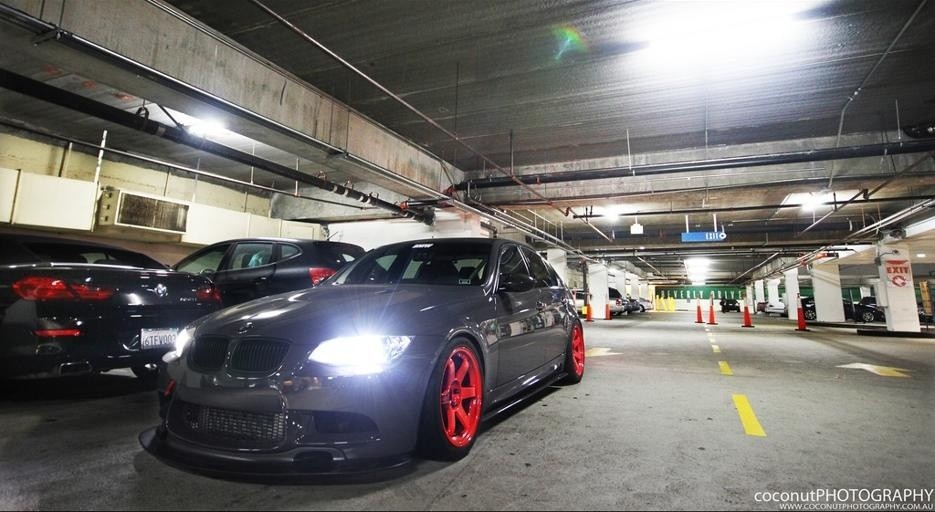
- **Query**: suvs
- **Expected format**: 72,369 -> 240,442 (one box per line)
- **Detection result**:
724,298 -> 740,313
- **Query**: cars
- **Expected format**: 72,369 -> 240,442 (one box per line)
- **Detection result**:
758,293 -> 935,323
568,288 -> 653,322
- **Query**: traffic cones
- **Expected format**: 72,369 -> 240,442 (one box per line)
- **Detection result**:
741,294 -> 755,328
794,293 -> 812,332
707,306 -> 718,325
693,300 -> 706,324
